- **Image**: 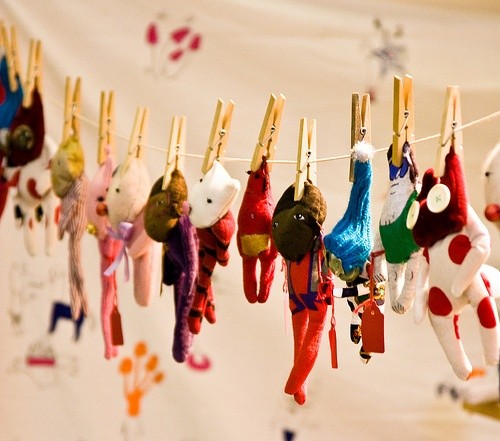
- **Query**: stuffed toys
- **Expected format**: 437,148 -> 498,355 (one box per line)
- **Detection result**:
1,55 -> 499,403
